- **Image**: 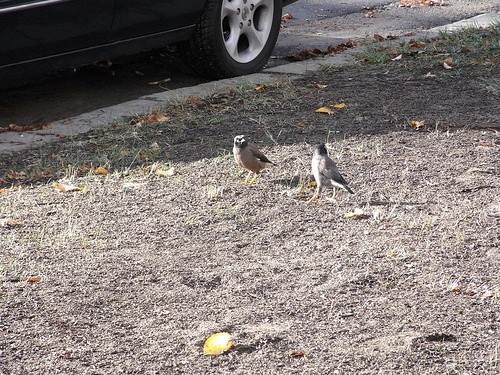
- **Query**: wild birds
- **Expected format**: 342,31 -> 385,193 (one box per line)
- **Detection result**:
302,141 -> 355,208
230,135 -> 272,186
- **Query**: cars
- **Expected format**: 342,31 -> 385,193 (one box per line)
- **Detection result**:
0,0 -> 301,79
